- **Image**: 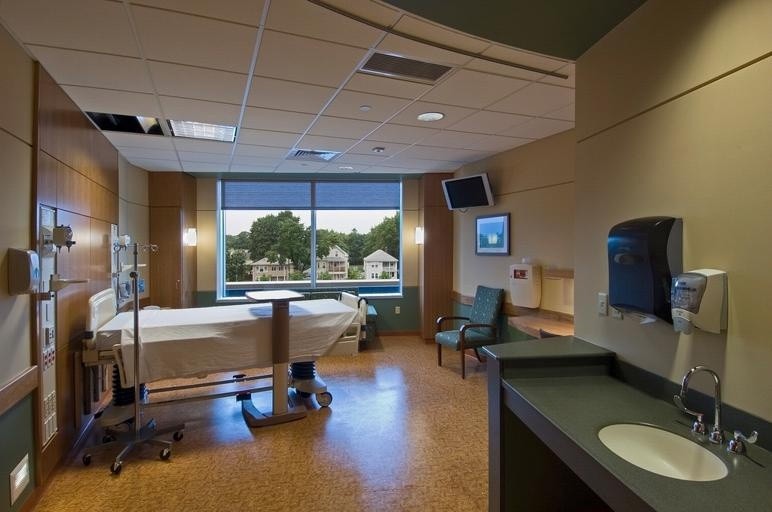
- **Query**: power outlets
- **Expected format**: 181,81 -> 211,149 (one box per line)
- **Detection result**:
395,306 -> 400,314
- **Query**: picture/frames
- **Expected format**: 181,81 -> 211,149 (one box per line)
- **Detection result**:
474,212 -> 511,256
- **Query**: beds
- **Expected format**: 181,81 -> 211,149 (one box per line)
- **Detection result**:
84,288 -> 369,444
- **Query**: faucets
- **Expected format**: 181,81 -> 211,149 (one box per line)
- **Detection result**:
681,366 -> 726,444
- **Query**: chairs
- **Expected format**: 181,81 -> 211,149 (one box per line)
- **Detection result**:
435,285 -> 504,379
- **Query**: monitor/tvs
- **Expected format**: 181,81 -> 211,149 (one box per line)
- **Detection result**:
442,173 -> 494,211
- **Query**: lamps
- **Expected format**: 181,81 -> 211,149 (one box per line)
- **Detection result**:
187,227 -> 197,247
415,226 -> 423,245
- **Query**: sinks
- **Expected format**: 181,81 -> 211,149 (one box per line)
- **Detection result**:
597,422 -> 729,482
